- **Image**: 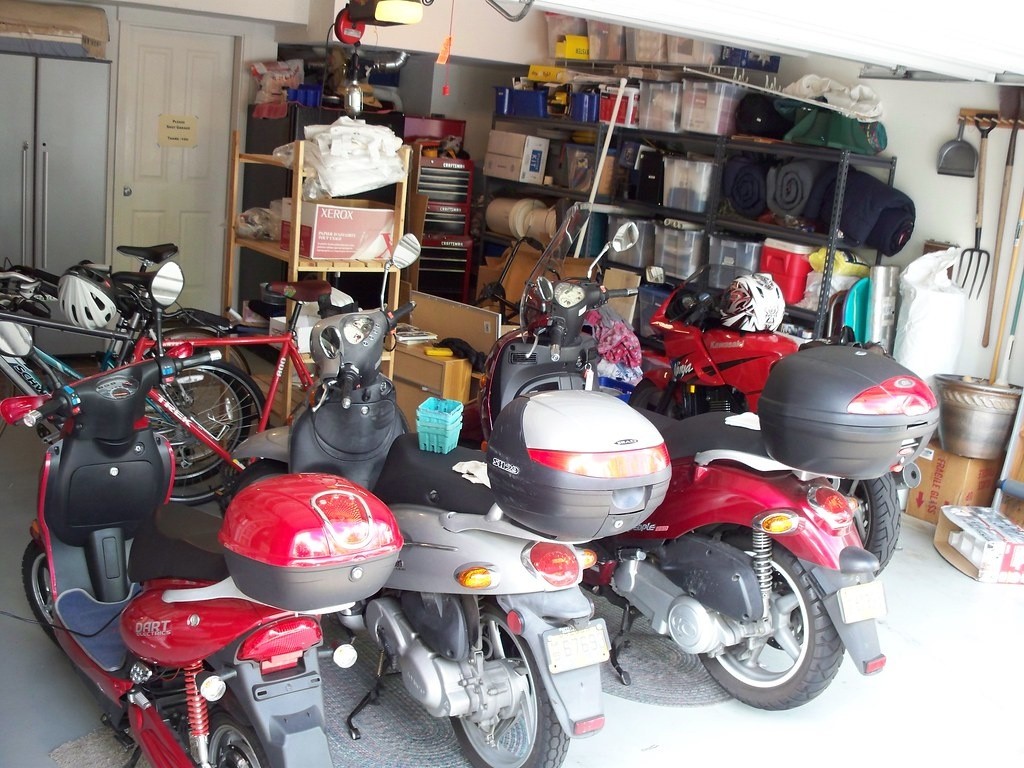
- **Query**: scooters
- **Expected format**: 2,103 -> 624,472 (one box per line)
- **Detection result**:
214,231 -> 674,768
1,260 -> 405,768
479,218 -> 940,715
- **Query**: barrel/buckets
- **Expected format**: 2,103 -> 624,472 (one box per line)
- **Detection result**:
935,373 -> 1022,459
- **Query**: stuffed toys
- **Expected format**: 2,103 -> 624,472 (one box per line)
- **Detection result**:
254,69 -> 293,105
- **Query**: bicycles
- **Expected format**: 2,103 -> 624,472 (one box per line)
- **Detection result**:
0,240 -> 272,509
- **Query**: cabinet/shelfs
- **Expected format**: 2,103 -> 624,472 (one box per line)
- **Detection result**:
468,108 -> 896,347
223,128 -> 410,341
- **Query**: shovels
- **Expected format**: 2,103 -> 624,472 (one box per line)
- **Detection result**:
938,117 -> 979,178
982,86 -> 1023,347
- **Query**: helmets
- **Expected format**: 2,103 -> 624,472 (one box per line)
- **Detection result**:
58,265 -> 117,330
717,272 -> 786,331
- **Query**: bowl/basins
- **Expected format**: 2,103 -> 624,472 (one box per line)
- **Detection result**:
322,95 -> 342,107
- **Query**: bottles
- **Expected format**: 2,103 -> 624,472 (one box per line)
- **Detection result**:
343,80 -> 363,117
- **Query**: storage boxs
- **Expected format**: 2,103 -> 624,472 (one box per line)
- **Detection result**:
759,344 -> 941,477
486,390 -> 673,541
933,504 -> 1024,585
903,442 -> 1009,523
482,10 -> 837,340
277,197 -> 396,260
217,471 -> 404,611
